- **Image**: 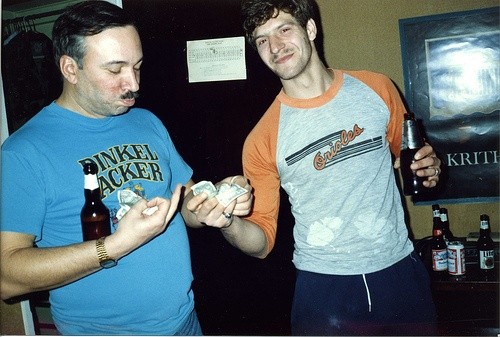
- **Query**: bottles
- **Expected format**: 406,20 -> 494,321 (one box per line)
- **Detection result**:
476,215 -> 495,279
431,204 -> 447,276
80,163 -> 111,242
400,112 -> 428,197
440,208 -> 453,248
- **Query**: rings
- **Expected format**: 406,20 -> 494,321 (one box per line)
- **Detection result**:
230,174 -> 241,187
223,211 -> 232,219
434,168 -> 439,176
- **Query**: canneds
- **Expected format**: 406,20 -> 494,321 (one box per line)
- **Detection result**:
447,241 -> 465,275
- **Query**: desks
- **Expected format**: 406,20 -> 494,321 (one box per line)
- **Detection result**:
428,259 -> 500,327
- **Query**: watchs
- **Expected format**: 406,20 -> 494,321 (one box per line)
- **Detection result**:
95,236 -> 117,269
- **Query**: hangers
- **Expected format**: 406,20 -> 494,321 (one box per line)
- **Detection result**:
2,14 -> 45,38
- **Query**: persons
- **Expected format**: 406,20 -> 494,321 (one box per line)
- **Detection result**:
0,0 -> 254,335
186,0 -> 441,336
